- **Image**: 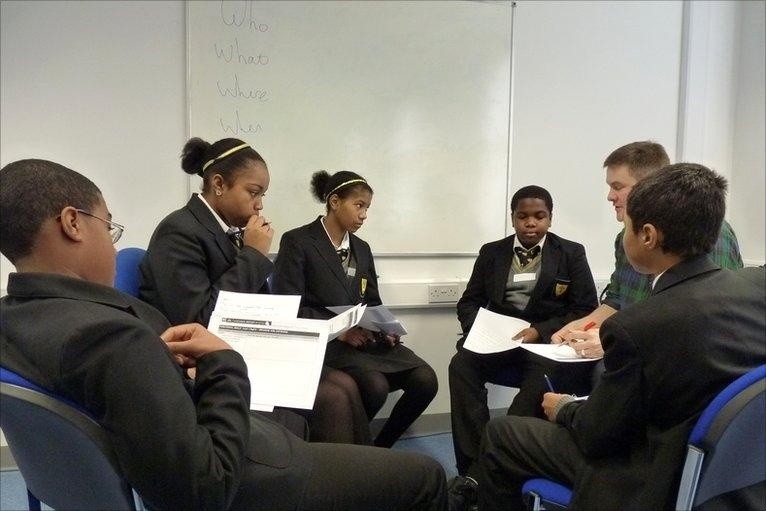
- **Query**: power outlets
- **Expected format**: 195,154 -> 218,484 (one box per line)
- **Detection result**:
427,283 -> 460,304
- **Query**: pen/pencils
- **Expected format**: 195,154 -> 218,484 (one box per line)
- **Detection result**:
377,341 -> 403,344
543,373 -> 555,393
558,321 -> 596,347
226,222 -> 271,235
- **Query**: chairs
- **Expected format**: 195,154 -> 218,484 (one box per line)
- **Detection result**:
1,367 -> 140,510
522,367 -> 766,511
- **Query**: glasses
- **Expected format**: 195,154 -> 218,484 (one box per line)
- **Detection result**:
57,207 -> 127,246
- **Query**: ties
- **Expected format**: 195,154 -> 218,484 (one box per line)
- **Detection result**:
514,247 -> 543,270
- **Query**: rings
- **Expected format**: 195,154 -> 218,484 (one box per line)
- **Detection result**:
581,349 -> 586,356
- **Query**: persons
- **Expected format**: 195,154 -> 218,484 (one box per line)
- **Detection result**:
550,142 -> 744,393
271,170 -> 437,448
448,186 -> 600,480
0,159 -> 447,511
470,161 -> 766,510
136,136 -> 375,448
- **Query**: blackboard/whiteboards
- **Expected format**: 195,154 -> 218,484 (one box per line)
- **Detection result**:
186,0 -> 516,257
